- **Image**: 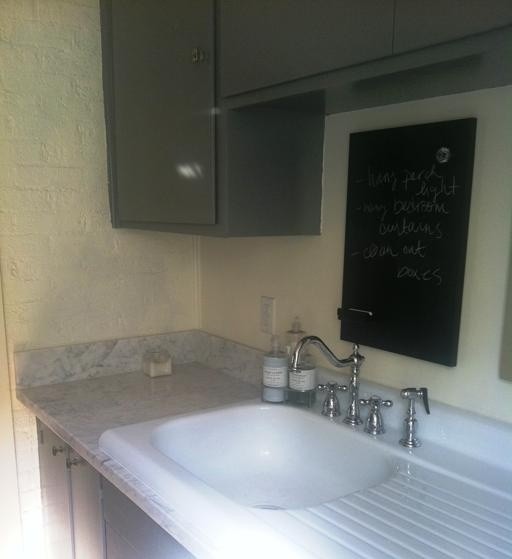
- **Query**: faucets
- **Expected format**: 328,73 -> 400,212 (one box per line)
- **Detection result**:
290,335 -> 365,425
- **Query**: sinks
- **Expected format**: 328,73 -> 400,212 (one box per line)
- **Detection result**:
98,397 -> 398,508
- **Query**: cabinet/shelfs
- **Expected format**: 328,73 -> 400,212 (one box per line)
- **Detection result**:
36,415 -> 192,559
98,1 -> 325,239
217,0 -> 512,109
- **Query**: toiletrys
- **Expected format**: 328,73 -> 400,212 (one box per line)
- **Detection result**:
264,314 -> 318,405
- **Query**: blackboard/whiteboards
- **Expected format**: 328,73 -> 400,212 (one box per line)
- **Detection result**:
337,116 -> 478,367
